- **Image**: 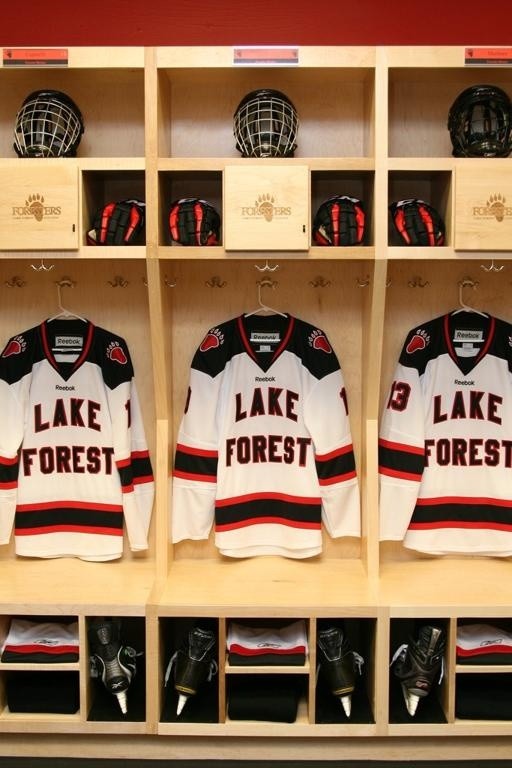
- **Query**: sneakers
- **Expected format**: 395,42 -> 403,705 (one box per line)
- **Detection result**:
316,628 -> 364,696
171,625 -> 218,697
88,621 -> 144,694
394,625 -> 447,697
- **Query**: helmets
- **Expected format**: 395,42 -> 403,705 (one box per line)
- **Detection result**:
13,89 -> 84,158
447,85 -> 512,157
233,89 -> 298,158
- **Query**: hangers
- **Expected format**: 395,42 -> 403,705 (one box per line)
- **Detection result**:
242,279 -> 288,343
450,280 -> 486,343
42,279 -> 87,352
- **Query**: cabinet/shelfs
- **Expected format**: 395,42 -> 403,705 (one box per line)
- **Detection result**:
0,42 -> 512,761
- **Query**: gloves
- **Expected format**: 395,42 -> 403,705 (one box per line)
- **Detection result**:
312,195 -> 368,246
388,198 -> 445,246
87,200 -> 146,246
168,197 -> 220,246
